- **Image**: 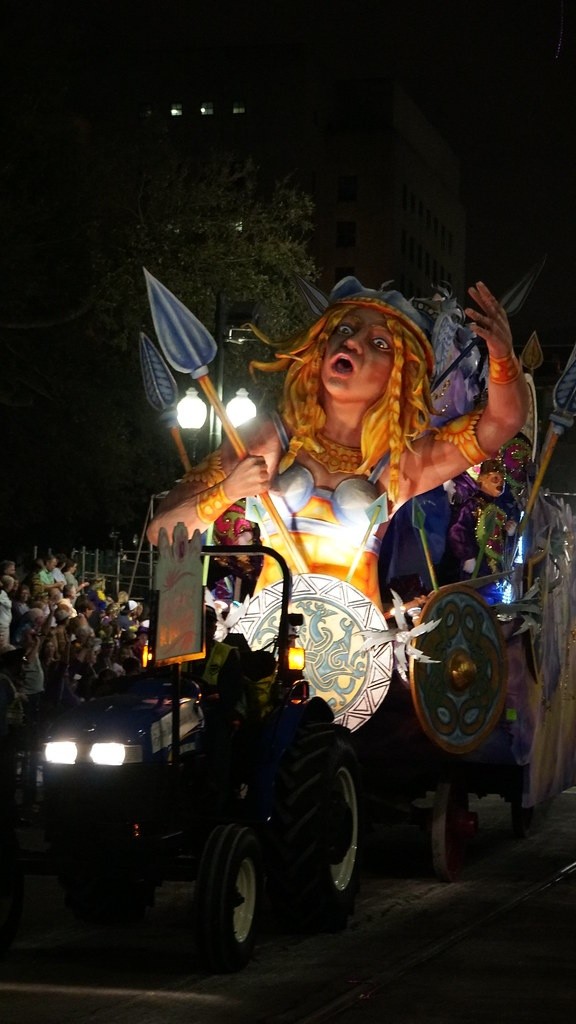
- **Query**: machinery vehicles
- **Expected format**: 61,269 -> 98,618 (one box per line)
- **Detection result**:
0,545 -> 366,974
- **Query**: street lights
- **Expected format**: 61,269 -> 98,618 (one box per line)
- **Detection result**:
172,387 -> 260,453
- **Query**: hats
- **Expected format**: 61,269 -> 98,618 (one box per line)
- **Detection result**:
205,605 -> 218,621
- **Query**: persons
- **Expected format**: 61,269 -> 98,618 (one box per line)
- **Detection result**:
448,458 -> 532,582
149,282 -> 528,671
1,552 -> 154,743
181,604 -> 248,745
